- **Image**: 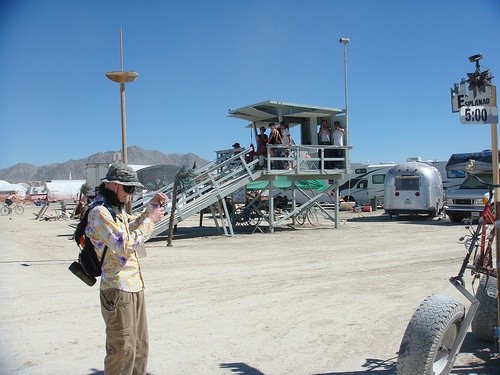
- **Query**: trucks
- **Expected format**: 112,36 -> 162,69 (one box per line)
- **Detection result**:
340,164 -> 398,205
445,149 -> 500,224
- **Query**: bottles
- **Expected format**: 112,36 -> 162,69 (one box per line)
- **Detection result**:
131,222 -> 147,259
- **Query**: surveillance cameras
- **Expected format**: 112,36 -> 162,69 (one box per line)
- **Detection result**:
469,54 -> 482,62
340,38 -> 350,43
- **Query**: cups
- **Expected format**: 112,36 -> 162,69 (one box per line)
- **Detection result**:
69,261 -> 97,286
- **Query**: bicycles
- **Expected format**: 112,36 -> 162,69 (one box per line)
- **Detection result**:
0,203 -> 25,216
296,208 -> 319,227
234,207 -> 260,227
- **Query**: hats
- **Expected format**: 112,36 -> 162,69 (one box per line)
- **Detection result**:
232,143 -> 240,146
99,163 -> 144,187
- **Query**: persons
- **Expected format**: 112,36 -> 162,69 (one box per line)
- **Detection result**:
76,198 -> 92,221
232,119 -> 345,170
222,194 -> 289,217
5,192 -> 21,214
34,196 -> 49,206
85,163 -> 170,375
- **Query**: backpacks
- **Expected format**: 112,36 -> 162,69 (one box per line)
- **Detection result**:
75,200 -> 116,278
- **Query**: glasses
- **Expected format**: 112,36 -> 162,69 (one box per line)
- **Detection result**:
123,186 -> 135,193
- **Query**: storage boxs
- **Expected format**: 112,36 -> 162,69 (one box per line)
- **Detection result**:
354,205 -> 372,213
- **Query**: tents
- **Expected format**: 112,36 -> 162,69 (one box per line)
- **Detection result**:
246,175 -> 336,213
